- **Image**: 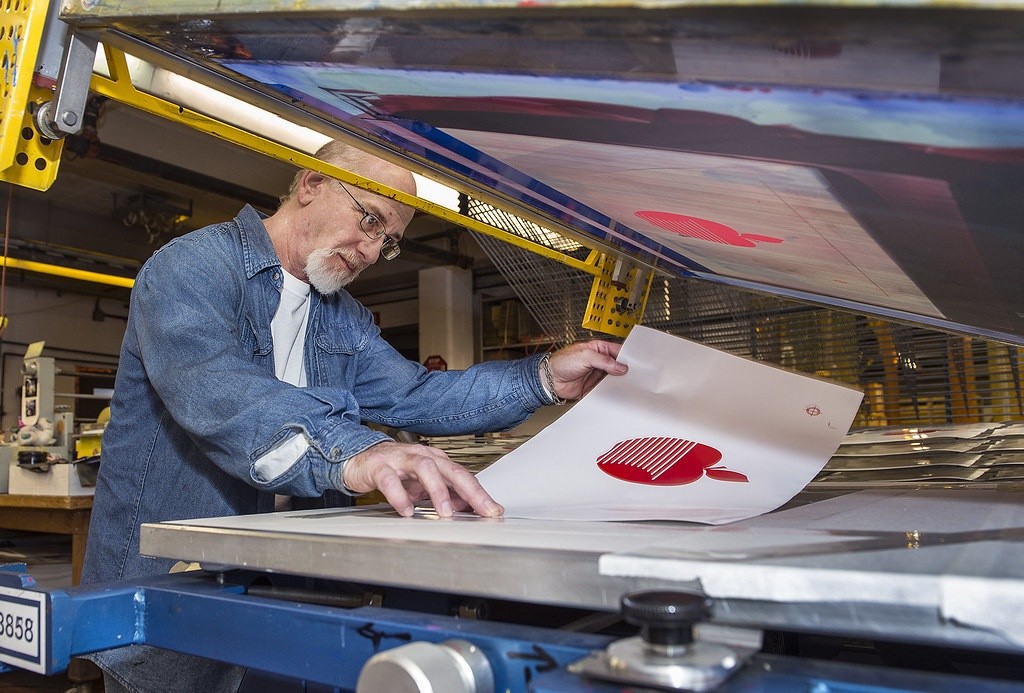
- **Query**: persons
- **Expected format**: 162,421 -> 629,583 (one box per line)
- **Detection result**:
73,140 -> 628,693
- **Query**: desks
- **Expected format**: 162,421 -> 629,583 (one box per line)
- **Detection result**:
0,492 -> 102,693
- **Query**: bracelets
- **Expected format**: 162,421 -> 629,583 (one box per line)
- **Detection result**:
543,351 -> 566,406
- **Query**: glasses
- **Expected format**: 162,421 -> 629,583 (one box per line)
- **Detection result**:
338,177 -> 403,264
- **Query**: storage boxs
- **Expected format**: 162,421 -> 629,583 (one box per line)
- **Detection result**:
8,461 -> 96,496
